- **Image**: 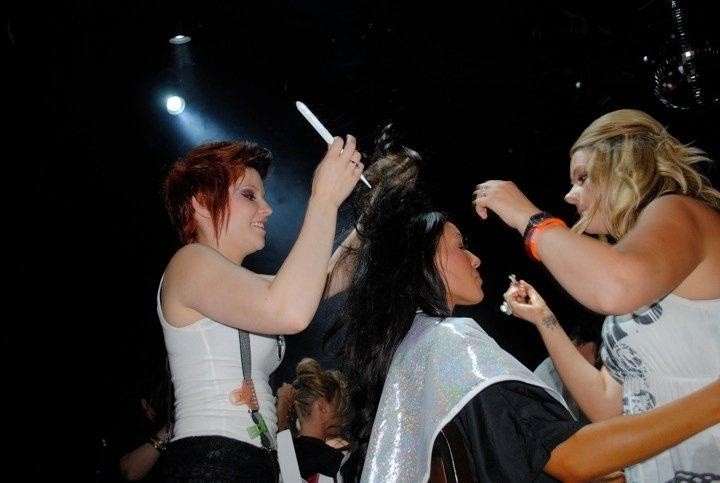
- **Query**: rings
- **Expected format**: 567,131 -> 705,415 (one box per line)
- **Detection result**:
349,160 -> 359,168
481,192 -> 487,197
501,302 -> 511,315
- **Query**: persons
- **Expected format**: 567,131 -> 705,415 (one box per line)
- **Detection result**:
532,299 -> 606,409
472,110 -> 720,483
118,375 -> 176,482
273,358 -> 354,483
96,108 -> 720,482
317,145 -> 720,482
156,132 -> 364,481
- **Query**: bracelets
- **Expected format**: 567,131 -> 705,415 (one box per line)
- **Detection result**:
152,434 -> 166,447
531,218 -> 566,261
148,438 -> 163,454
